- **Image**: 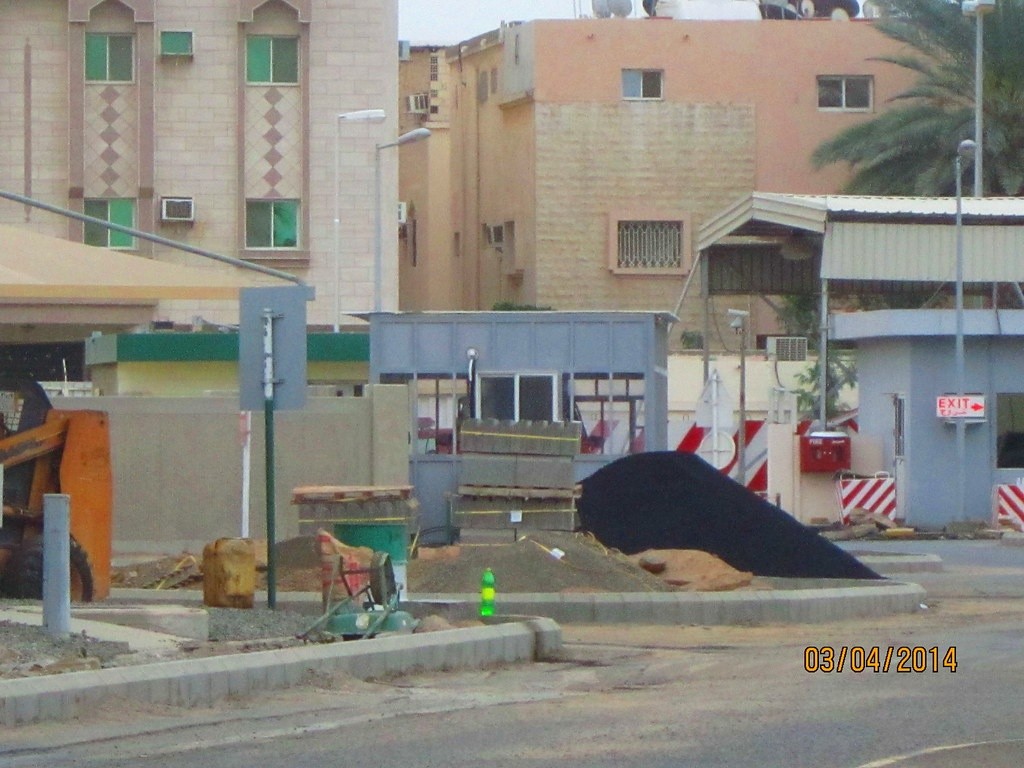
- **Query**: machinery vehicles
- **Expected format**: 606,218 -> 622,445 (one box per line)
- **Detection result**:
0,408 -> 115,605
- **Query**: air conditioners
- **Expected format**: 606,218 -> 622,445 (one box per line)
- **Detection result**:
160,198 -> 194,222
484,223 -> 503,244
398,202 -> 407,224
406,95 -> 429,112
399,41 -> 410,58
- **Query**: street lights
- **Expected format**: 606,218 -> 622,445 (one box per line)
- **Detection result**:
375,128 -> 432,310
334,108 -> 387,333
954,137 -> 979,530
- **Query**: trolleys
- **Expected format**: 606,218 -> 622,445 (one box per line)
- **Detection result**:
296,549 -> 418,649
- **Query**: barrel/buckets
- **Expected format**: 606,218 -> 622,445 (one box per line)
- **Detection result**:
329,523 -> 408,634
409,454 -> 460,544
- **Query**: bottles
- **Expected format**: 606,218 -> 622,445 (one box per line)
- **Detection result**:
480,567 -> 495,616
